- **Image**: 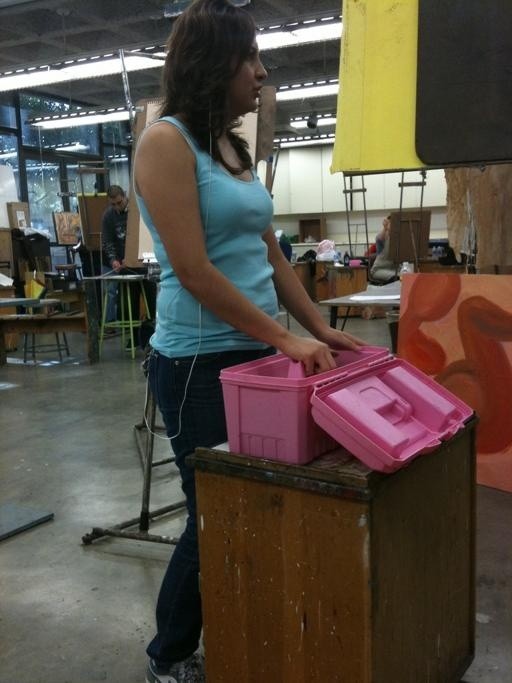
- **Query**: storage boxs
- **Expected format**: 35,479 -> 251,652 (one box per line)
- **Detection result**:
218,348 -> 392,466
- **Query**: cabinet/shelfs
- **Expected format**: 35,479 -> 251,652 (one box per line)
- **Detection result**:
271,144 -> 448,217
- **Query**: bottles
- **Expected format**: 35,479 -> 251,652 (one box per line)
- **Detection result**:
400,261 -> 411,282
344,252 -> 349,265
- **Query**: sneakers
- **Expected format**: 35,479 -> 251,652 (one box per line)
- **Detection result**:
125,338 -> 142,351
143,655 -> 205,683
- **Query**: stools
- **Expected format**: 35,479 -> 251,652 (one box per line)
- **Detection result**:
21,296 -> 71,361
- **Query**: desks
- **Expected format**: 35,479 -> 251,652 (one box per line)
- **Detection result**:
320,290 -> 404,352
184,414 -> 475,679
82,274 -> 152,359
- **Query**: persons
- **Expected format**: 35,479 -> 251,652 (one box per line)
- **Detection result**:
370,215 -> 401,284
77,229 -> 118,337
101,183 -> 157,352
130,0 -> 370,683
